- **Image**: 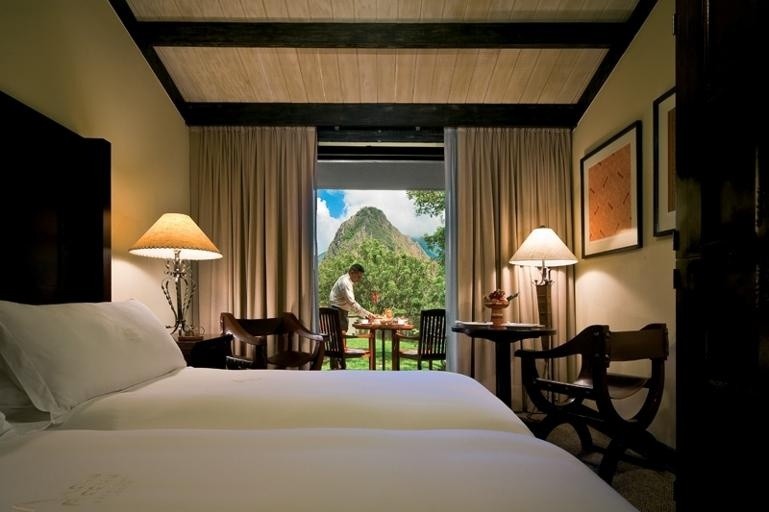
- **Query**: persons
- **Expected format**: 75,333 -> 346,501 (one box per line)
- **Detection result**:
330,264 -> 376,347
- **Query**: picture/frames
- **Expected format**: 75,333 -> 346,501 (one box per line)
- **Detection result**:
580,119 -> 643,259
653,86 -> 677,236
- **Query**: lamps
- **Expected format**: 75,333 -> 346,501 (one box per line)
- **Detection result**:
129,213 -> 223,340
509,225 -> 578,418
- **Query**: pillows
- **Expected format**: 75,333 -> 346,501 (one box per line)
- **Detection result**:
0,299 -> 187,427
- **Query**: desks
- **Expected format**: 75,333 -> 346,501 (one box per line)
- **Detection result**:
451,322 -> 557,411
353,321 -> 415,370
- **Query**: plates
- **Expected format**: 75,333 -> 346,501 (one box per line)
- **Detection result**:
454,320 -> 541,330
177,335 -> 203,341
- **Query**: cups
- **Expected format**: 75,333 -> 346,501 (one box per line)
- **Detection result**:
376,316 -> 398,324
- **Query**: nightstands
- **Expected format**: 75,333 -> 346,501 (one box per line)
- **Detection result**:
171,333 -> 234,369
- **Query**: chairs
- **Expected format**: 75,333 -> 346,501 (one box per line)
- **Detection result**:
394,309 -> 446,370
319,308 -> 372,369
514,323 -> 675,486
220,312 -> 329,370
